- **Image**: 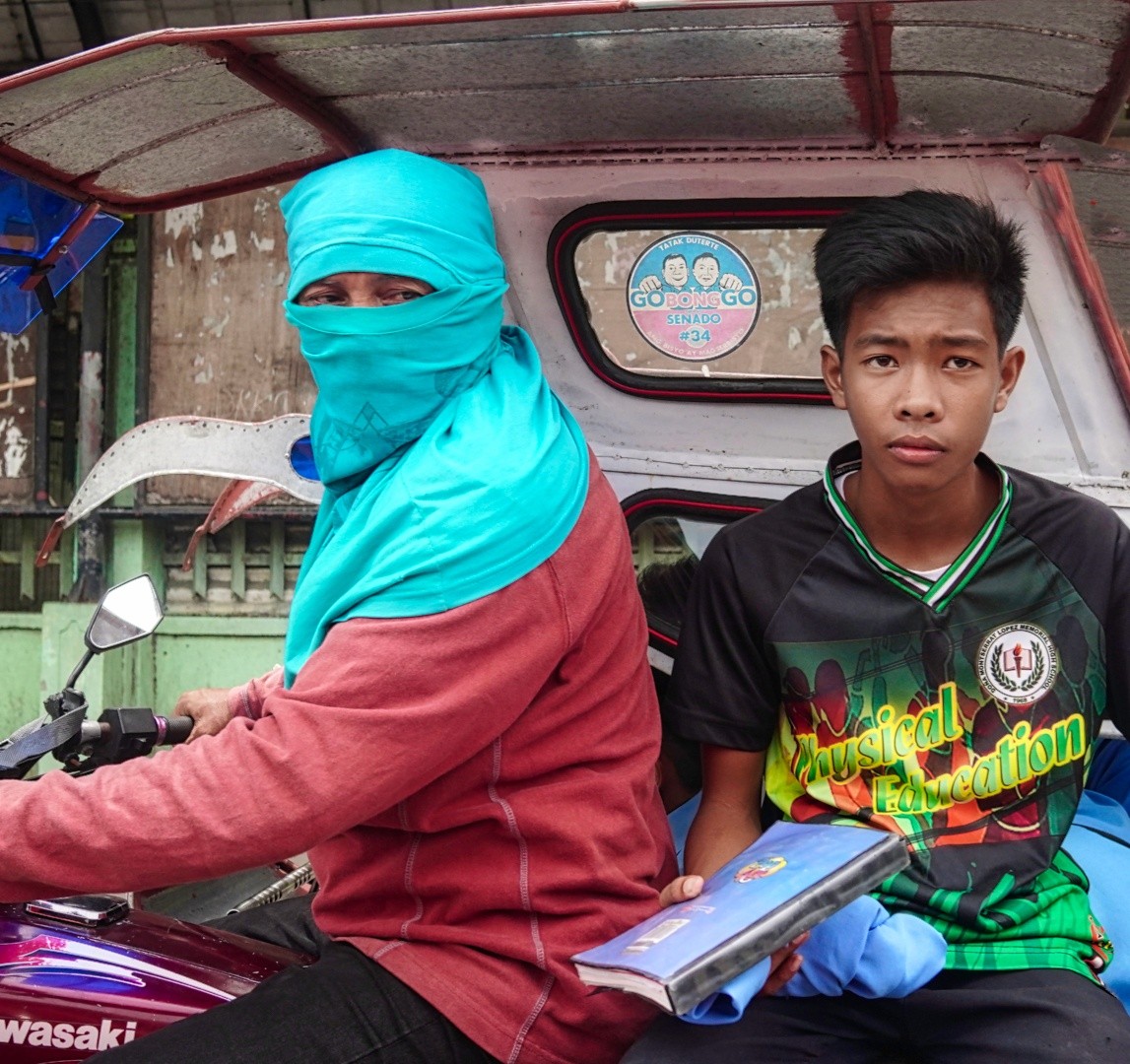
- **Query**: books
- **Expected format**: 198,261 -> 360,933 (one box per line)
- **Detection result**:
569,820 -> 911,1017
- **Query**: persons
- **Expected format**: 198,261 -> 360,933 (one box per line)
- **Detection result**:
611,189 -> 1129,1064
0,150 -> 679,1064
